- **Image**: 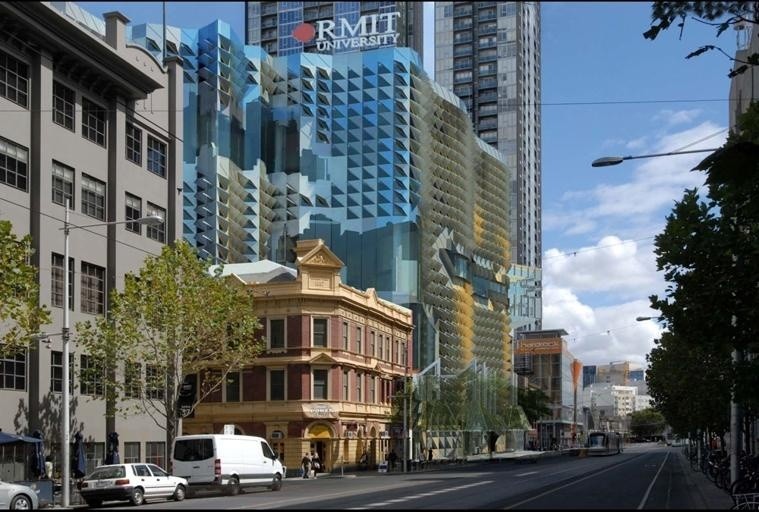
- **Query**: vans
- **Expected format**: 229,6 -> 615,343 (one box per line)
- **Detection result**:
169,434 -> 284,496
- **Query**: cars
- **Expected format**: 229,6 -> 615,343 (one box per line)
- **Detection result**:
80,462 -> 188,507
0,478 -> 39,510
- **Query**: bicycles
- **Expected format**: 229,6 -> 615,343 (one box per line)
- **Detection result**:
682,444 -> 759,512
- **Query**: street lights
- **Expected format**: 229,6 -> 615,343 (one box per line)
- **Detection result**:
636,314 -> 666,325
589,142 -> 743,496
62,213 -> 163,508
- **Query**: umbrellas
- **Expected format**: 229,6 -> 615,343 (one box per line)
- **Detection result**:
0,428 -> 46,446
29,431 -> 47,483
104,430 -> 122,464
70,429 -> 87,503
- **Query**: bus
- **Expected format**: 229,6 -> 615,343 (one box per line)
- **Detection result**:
588,431 -> 623,456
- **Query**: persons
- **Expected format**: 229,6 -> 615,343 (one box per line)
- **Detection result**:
43,455 -> 54,478
300,452 -> 311,479
426,447 -> 433,462
359,451 -> 369,465
390,449 -> 400,469
311,451 -> 323,479
